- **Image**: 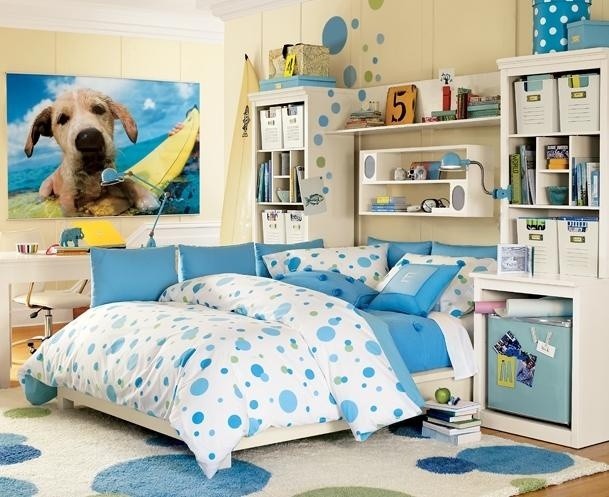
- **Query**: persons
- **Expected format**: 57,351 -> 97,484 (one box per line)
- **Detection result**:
168,122 -> 200,157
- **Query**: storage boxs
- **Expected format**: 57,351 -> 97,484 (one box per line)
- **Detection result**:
261,212 -> 285,243
259,108 -> 282,150
281,105 -> 304,148
513,79 -> 557,132
558,220 -> 599,278
285,212 -> 307,243
557,75 -> 600,132
516,218 -> 559,277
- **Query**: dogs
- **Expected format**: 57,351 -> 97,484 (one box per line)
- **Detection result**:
23,88 -> 162,217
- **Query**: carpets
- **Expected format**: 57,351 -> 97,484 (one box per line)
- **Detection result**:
0,383 -> 609,497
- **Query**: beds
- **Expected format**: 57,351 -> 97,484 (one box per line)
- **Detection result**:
18,235 -> 498,480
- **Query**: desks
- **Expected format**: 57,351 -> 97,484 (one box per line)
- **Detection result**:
0,250 -> 91,388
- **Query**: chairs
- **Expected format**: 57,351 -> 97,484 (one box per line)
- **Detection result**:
13,280 -> 90,355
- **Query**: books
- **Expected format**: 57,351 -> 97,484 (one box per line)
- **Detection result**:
420,397 -> 482,446
370,195 -> 409,212
345,111 -> 383,128
257,159 -> 272,202
509,144 -> 535,205
571,157 -> 599,207
466,95 -> 501,117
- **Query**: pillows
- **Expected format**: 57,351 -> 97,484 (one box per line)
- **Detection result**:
89,244 -> 178,306
262,243 -> 389,289
177,242 -> 256,280
255,239 -> 324,277
369,263 -> 463,318
376,252 -> 497,319
274,270 -> 380,308
431,240 -> 497,260
366,236 -> 432,271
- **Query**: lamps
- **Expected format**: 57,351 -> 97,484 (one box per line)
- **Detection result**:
441,151 -> 511,202
99,168 -> 170,247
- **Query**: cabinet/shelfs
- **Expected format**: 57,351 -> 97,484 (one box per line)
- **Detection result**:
326,115 -> 500,135
358,144 -> 494,219
468,47 -> 609,449
247,85 -> 355,248
